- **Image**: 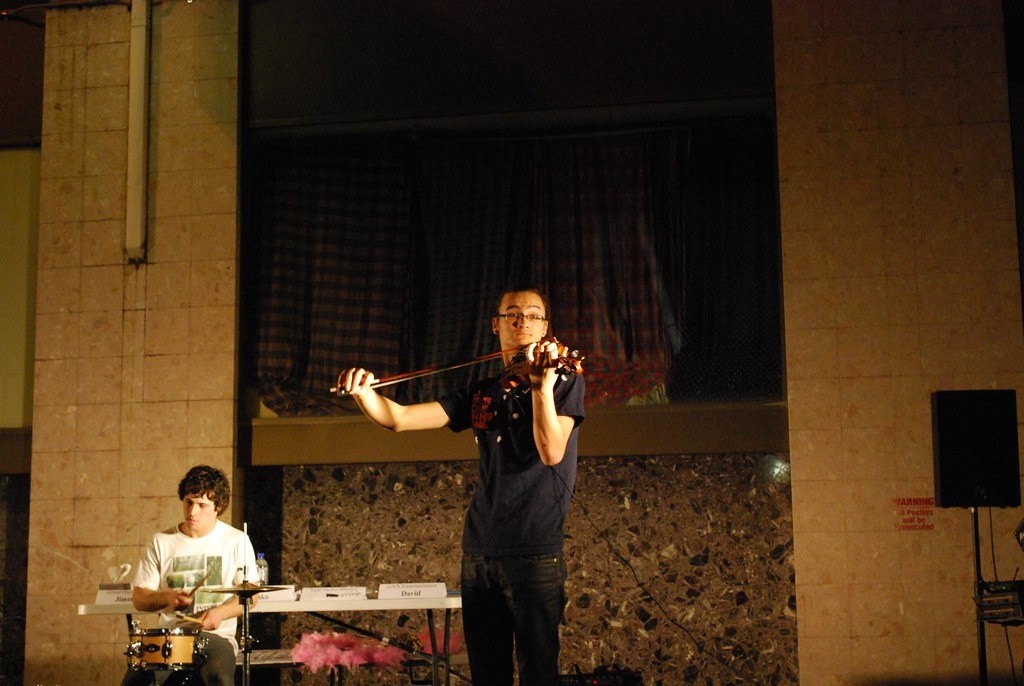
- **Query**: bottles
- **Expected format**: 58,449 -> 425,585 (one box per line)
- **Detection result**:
256,553 -> 269,585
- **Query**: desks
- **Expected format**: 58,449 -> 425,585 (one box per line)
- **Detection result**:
78,596 -> 462,686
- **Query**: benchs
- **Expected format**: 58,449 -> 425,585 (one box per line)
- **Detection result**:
234,649 -> 469,665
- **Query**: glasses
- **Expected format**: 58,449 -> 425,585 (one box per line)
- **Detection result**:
499,312 -> 545,324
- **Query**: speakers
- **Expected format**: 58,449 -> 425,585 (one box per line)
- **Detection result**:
929,390 -> 1021,507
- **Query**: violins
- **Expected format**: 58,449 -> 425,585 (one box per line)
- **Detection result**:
496,337 -> 585,393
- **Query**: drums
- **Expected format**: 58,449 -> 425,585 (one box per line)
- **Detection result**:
128,628 -> 200,671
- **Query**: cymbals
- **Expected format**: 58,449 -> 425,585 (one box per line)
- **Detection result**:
200,585 -> 287,595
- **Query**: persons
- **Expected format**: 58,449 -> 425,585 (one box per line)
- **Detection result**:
335,283 -> 587,686
121,465 -> 259,686
